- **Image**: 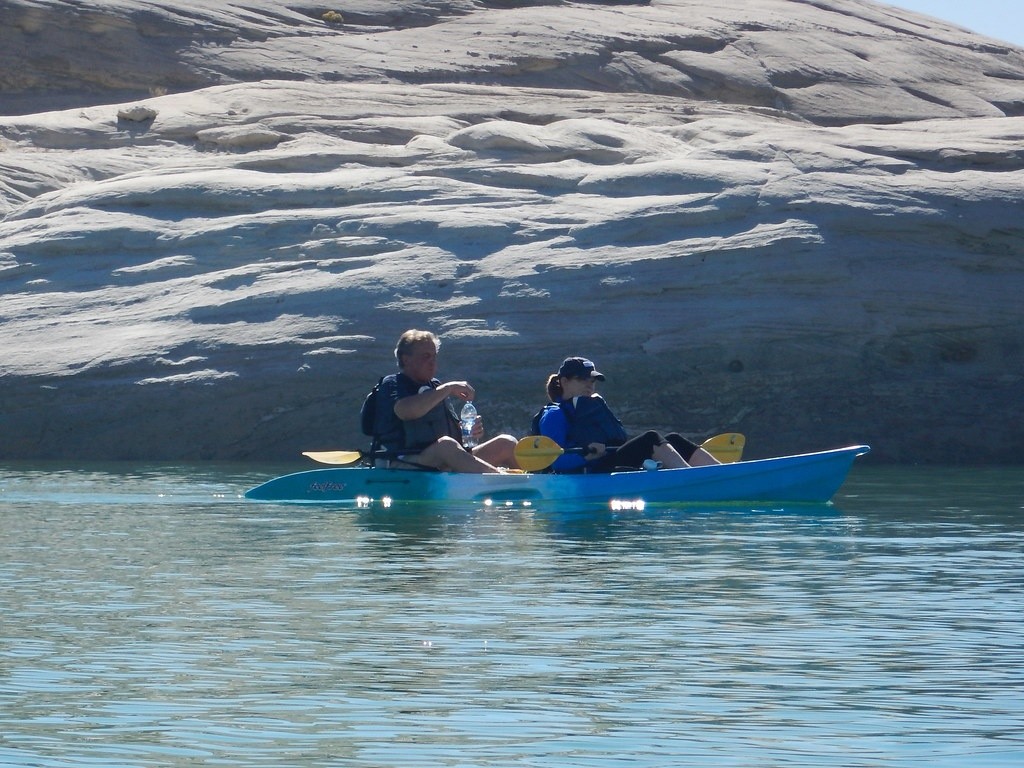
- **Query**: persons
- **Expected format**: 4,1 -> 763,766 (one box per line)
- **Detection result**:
528,357 -> 721,474
359,329 -> 522,474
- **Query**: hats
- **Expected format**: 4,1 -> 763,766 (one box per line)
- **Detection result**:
557,356 -> 606,382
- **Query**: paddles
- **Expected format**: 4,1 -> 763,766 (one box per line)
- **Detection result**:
303,449 -> 377,466
514,431 -> 748,472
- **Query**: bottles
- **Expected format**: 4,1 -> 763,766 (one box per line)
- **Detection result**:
460,400 -> 479,448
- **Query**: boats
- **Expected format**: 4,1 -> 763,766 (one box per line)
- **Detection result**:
244,438 -> 880,517
